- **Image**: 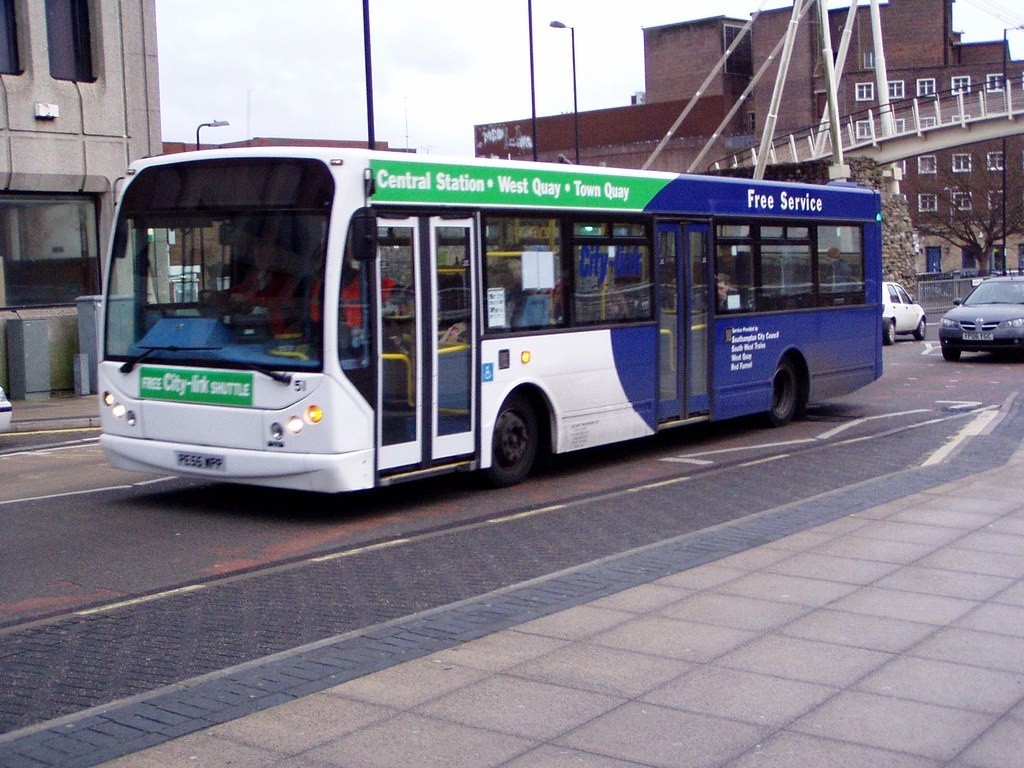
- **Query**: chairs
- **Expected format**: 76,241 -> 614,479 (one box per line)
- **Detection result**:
512,295 -> 552,327
661,255 -> 836,312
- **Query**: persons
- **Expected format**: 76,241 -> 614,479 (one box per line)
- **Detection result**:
202,241 -> 367,354
821,247 -> 850,283
716,244 -> 750,312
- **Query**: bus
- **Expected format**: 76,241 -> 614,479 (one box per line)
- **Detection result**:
91,141 -> 885,495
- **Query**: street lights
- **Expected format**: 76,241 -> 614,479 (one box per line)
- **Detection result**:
195,120 -> 231,291
549,19 -> 581,164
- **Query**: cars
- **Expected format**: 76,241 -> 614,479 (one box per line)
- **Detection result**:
882,281 -> 927,346
938,276 -> 1024,361
